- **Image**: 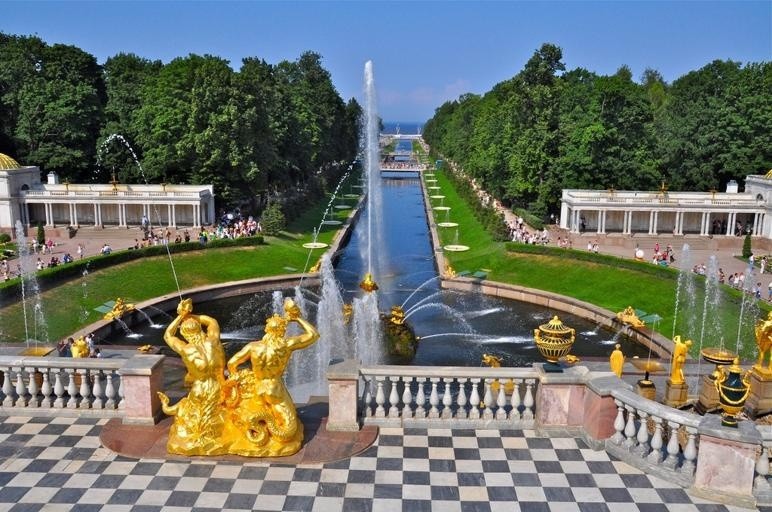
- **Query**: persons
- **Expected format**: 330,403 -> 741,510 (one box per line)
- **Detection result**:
0,205 -> 263,282
500,210 -> 599,254
691,253 -> 771,305
56,332 -> 103,358
651,242 -> 676,267
609,343 -> 627,379
227,302 -> 322,406
753,310 -> 771,372
711,218 -> 754,237
482,353 -> 500,368
113,296 -> 126,312
671,335 -> 693,385
158,299 -> 227,418
